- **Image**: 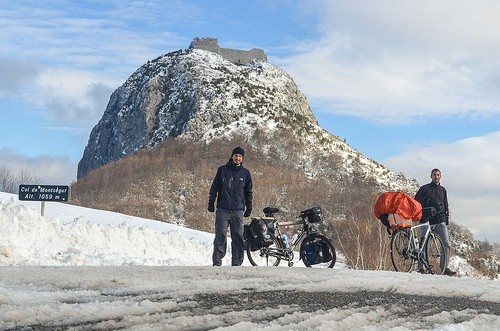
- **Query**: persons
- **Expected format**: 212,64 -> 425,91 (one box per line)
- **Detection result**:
208,146 -> 253,266
414,168 -> 456,277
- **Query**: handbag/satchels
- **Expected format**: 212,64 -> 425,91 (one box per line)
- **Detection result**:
243,224 -> 259,250
251,219 -> 275,246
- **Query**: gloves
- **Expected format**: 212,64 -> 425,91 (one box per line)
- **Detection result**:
244,209 -> 251,217
208,200 -> 214,212
446,216 -> 449,225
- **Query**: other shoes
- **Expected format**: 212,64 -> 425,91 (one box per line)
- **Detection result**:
420,270 -> 428,274
443,268 -> 456,276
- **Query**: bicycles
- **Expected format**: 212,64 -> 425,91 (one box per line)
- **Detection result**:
243,207 -> 336,268
391,207 -> 447,275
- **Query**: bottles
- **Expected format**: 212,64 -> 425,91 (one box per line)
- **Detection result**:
415,237 -> 419,249
290,233 -> 298,245
419,236 -> 425,248
281,233 -> 290,248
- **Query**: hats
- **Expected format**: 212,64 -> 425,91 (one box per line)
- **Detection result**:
233,147 -> 244,156
